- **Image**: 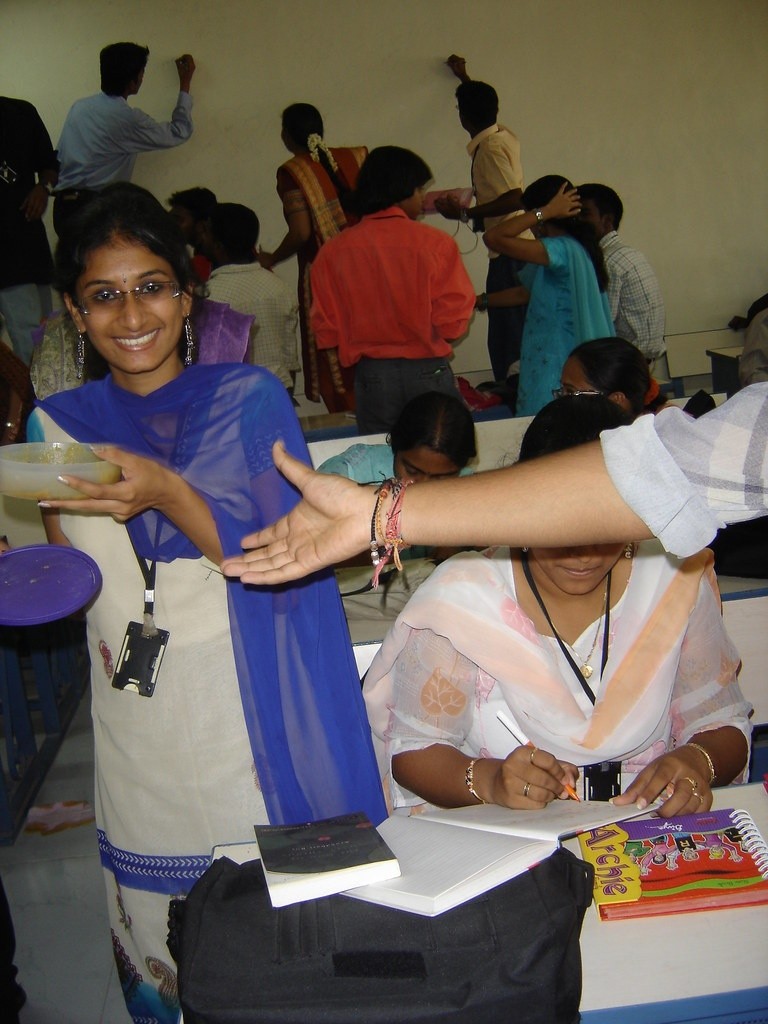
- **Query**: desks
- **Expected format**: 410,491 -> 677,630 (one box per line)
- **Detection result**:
704,345 -> 745,400
177,780 -> 768,1024
335,545 -> 768,641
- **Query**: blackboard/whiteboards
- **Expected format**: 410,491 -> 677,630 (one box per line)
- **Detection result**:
0,0 -> 768,418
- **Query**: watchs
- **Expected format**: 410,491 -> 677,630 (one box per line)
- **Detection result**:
458,207 -> 469,223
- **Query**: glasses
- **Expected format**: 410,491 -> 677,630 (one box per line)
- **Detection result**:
552,388 -> 602,399
76,281 -> 186,315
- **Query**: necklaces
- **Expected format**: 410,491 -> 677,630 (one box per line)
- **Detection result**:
550,575 -> 607,678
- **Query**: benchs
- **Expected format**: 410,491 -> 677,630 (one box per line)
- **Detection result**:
663,327 -> 748,397
304,393 -> 730,475
352,586 -> 768,786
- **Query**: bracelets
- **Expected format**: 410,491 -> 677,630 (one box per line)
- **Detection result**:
464,758 -> 489,805
532,208 -> 544,224
357,471 -> 415,589
477,292 -> 488,311
687,742 -> 716,785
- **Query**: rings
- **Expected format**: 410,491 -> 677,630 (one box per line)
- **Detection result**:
531,748 -> 540,759
692,792 -> 704,804
523,783 -> 531,796
684,776 -> 697,790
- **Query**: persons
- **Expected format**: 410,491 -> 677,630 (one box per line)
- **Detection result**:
317,390 -> 490,562
362,393 -> 755,818
0,41 -> 667,435
552,337 -> 693,427
219,380 -> 768,585
26,190 -> 393,1024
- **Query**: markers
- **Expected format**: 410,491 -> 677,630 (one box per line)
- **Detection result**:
444,61 -> 466,64
179,57 -> 187,65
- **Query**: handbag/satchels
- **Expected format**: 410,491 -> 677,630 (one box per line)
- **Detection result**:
168,847 -> 593,1024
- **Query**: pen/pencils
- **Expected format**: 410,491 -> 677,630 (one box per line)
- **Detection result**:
496,712 -> 581,802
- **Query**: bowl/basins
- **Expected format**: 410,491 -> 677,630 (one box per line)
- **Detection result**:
0,443 -> 121,501
